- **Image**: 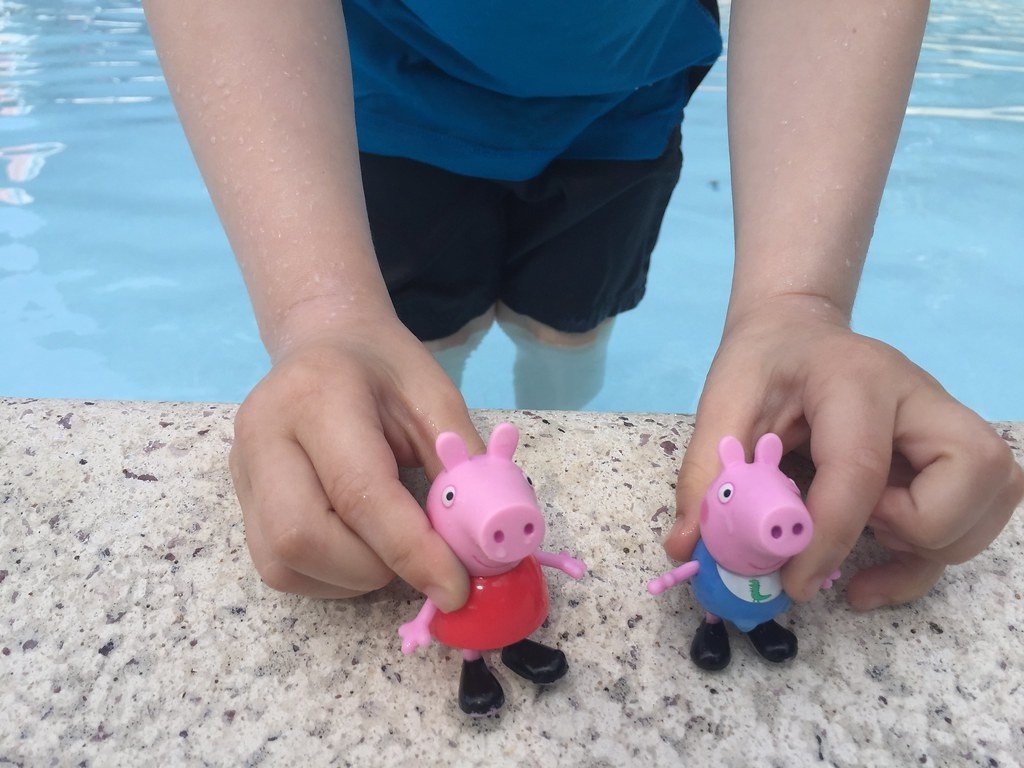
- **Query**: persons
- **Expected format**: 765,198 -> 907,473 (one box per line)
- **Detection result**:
142,0 -> 1024,611
